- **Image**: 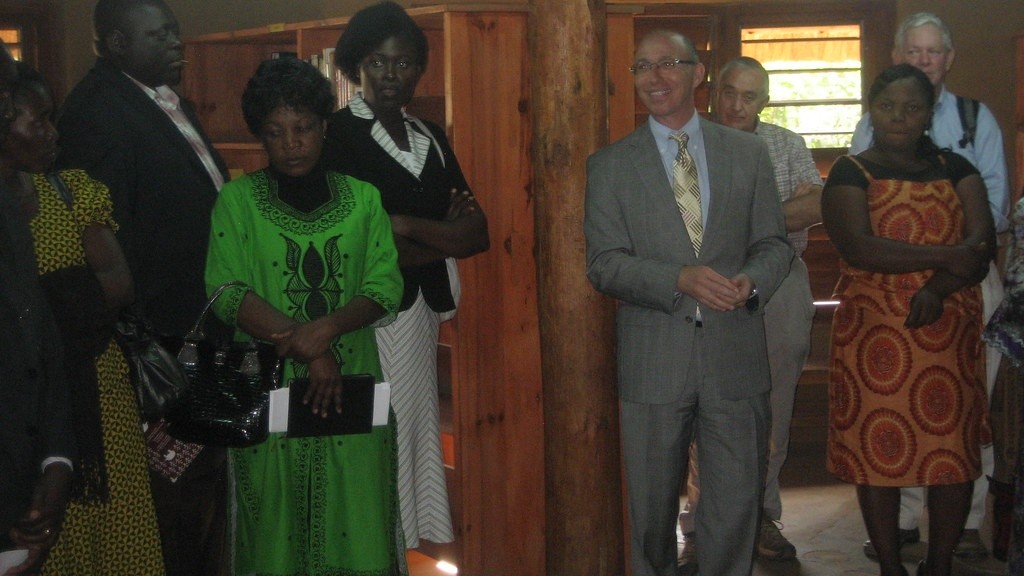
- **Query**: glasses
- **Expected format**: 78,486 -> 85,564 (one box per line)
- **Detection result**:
629,55 -> 695,76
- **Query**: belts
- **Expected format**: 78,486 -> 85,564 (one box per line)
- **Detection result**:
695,320 -> 703,329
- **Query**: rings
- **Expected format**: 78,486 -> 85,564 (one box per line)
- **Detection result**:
42,527 -> 52,535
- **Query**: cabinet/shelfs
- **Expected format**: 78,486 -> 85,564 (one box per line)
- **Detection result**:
180,3 -> 644,576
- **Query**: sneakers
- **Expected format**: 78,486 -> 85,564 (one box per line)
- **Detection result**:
676,533 -> 698,575
756,517 -> 797,561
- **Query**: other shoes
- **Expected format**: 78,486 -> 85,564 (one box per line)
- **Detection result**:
862,527 -> 918,556
953,529 -> 987,557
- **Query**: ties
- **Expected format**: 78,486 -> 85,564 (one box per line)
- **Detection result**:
667,131 -> 704,258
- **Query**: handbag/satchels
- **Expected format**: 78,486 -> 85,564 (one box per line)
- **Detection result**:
165,282 -> 270,448
114,315 -> 188,421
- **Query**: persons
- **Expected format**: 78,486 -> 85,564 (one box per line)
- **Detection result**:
0,0 -> 491,576
583,10 -> 1024,576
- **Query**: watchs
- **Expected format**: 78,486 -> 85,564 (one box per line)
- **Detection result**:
749,288 -> 759,300
